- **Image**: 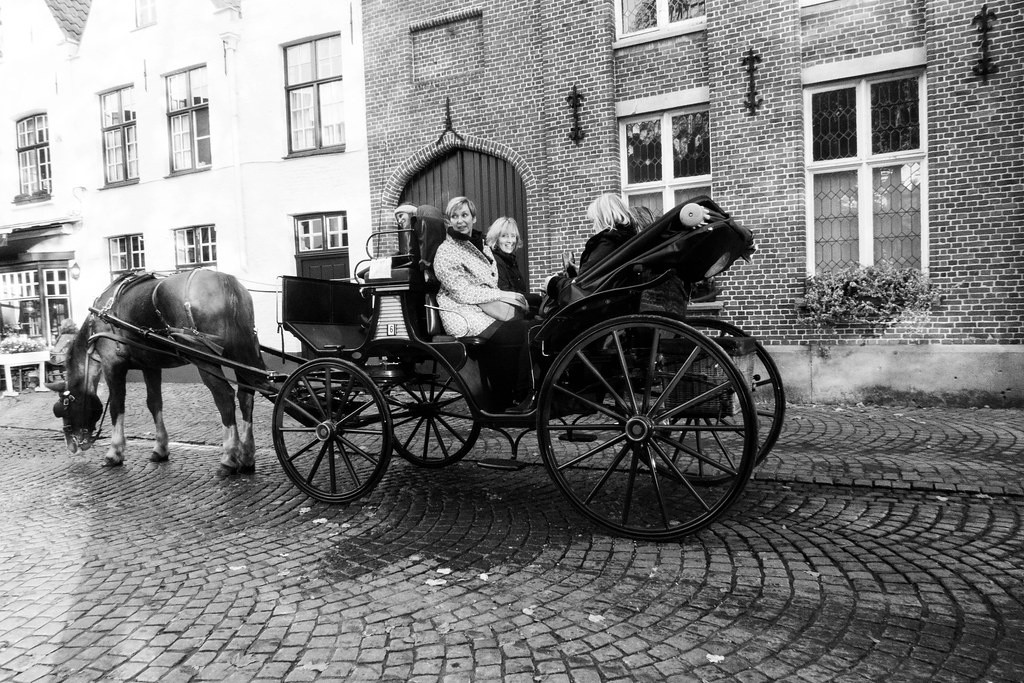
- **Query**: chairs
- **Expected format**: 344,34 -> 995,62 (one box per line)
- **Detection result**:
46,340 -> 75,383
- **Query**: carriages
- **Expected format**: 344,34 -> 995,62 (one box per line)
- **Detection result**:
41,194 -> 786,545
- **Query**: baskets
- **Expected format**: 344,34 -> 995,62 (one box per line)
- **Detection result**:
657,336 -> 757,417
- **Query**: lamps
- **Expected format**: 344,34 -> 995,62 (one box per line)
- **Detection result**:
70,263 -> 80,279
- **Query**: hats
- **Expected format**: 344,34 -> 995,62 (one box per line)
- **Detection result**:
394,202 -> 419,220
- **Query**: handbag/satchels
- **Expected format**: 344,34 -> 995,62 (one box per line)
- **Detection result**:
478,282 -> 526,322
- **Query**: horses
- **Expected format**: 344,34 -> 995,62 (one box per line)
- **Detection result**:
45,268 -> 269,477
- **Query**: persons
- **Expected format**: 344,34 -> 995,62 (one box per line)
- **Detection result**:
393,202 -> 447,268
486,216 -> 544,323
432,195 -> 533,405
503,192 -> 637,414
47,317 -> 79,383
629,202 -> 656,232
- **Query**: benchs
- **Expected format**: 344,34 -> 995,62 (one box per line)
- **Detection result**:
355,217 -> 488,344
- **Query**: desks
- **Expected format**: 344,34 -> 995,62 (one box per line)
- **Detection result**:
0,351 -> 50,397
